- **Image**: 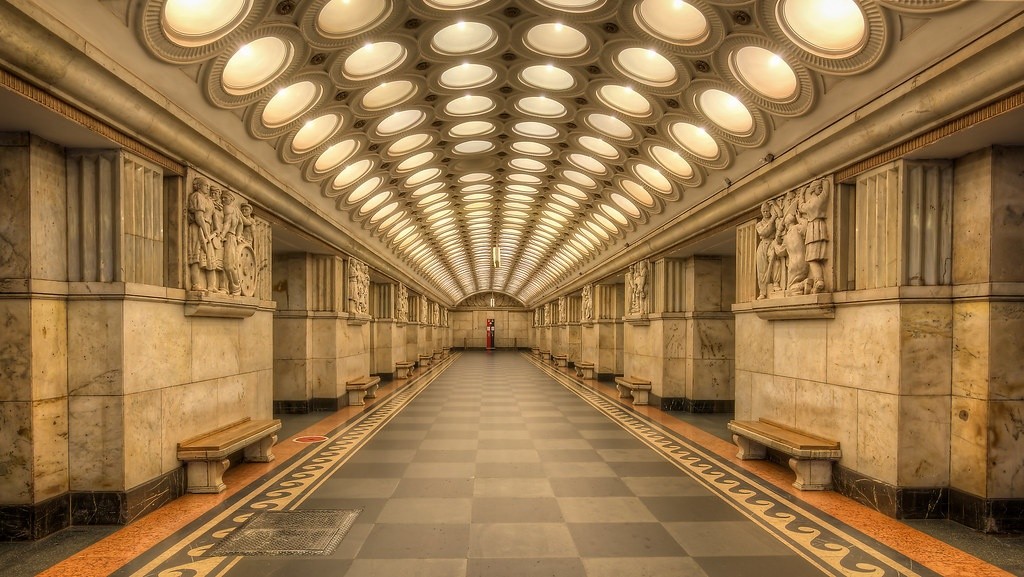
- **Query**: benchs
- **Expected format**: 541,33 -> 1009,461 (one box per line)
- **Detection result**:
540,350 -> 550,359
443,345 -> 454,354
434,351 -> 443,359
615,376 -> 652,405
574,361 -> 594,379
420,354 -> 433,366
531,346 -> 539,354
727,417 -> 842,490
553,353 -> 567,366
396,361 -> 415,378
346,375 -> 380,405
176,417 -> 282,493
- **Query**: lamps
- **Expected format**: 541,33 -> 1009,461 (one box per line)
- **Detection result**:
134,0 -> 968,307
